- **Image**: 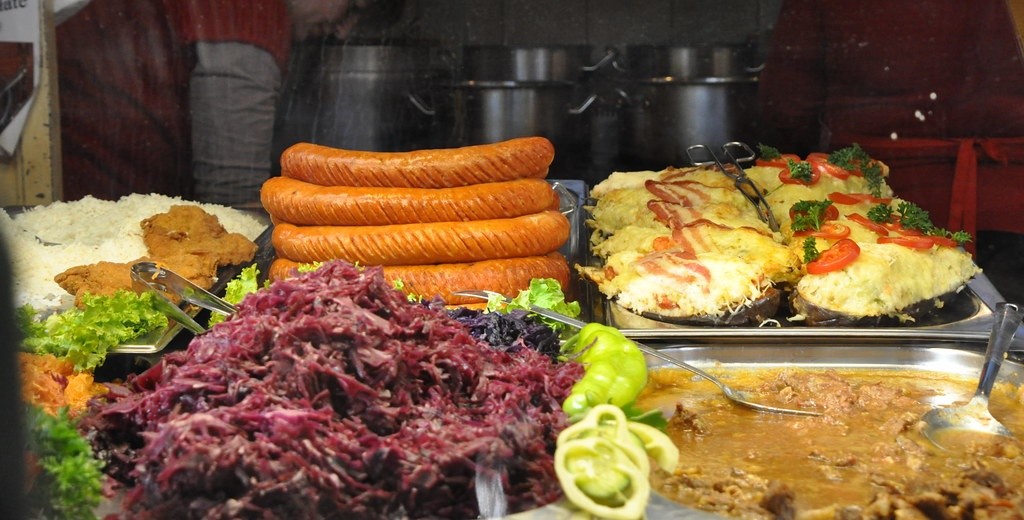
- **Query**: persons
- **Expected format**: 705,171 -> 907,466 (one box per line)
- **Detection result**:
56,0 -> 407,220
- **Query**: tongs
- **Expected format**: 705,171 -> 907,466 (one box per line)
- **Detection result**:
685,139 -> 787,243
128,261 -> 245,338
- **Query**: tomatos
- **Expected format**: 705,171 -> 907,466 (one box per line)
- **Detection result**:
754,152 -> 958,274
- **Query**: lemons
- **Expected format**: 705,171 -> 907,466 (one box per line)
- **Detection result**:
554,406 -> 681,520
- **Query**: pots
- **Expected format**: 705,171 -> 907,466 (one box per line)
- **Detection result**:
603,37 -> 776,80
290,38 -> 436,73
269,69 -> 441,177
596,73 -> 762,176
435,39 -> 618,82
401,77 -> 599,147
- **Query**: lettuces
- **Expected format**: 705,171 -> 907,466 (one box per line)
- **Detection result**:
0,292 -> 167,519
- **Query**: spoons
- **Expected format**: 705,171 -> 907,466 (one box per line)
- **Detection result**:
454,289 -> 829,416
919,302 -> 1024,455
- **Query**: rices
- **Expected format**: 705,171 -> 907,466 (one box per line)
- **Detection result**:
0,193 -> 267,312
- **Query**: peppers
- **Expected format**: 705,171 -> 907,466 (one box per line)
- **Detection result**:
556,324 -> 647,410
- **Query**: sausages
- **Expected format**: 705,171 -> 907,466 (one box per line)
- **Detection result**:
261,136 -> 572,312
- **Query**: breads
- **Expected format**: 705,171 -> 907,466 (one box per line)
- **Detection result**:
586,164 -> 792,308
